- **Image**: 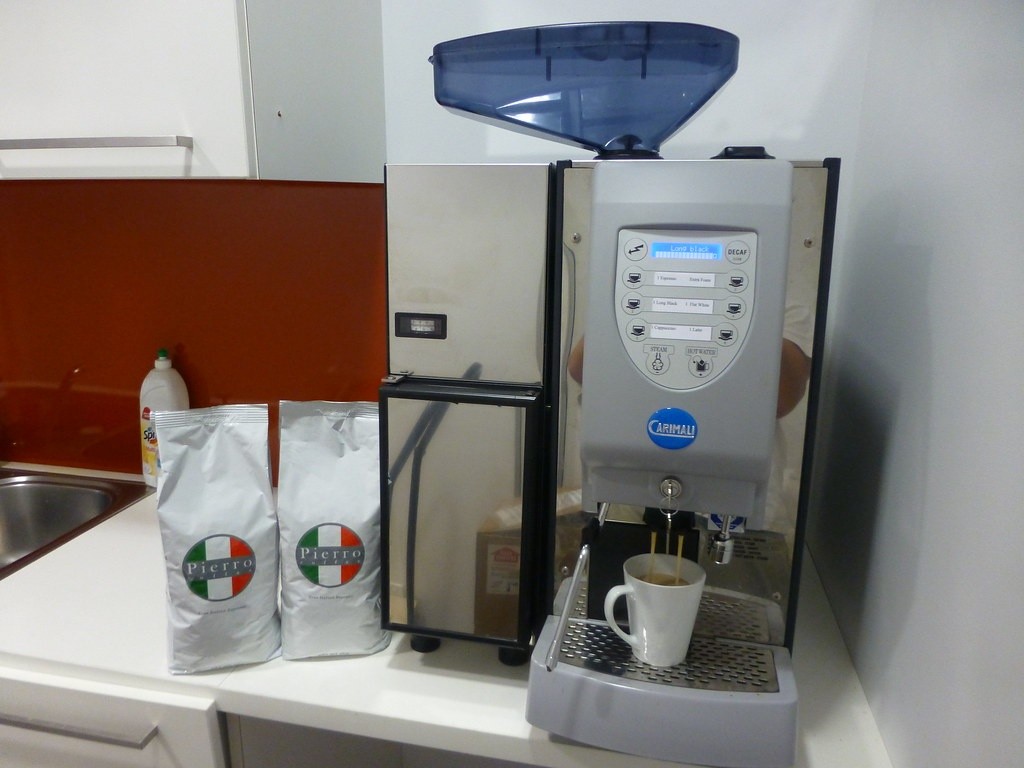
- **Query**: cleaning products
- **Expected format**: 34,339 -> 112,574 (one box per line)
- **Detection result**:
137,345 -> 191,489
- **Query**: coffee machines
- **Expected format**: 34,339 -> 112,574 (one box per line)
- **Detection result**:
378,21 -> 842,768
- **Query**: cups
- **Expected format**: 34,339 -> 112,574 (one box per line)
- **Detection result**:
605,552 -> 708,666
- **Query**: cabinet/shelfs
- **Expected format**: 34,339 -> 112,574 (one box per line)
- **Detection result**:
0,0 -> 386,186
0,664 -> 228,768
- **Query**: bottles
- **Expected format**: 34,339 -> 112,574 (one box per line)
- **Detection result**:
138,347 -> 191,488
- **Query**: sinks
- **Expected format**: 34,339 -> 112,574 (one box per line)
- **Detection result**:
1,467 -> 159,582
